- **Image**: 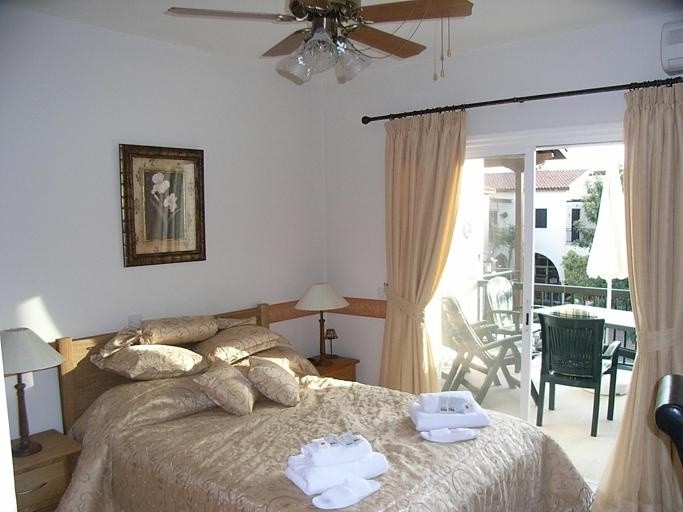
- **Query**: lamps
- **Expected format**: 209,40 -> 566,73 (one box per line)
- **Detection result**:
0,327 -> 66,457
277,27 -> 371,85
294,283 -> 350,367
326,329 -> 338,359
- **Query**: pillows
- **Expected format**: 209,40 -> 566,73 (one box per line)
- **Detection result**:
89,316 -> 300,416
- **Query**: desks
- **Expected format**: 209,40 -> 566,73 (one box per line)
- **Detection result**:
533,304 -> 635,329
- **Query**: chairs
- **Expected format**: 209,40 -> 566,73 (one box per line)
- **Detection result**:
441,277 -> 621,437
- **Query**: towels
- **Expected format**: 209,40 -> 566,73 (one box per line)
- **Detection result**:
302,435 -> 373,466
284,451 -> 389,496
417,391 -> 476,414
409,408 -> 490,431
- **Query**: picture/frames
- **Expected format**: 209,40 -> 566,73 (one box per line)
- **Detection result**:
119,144 -> 206,267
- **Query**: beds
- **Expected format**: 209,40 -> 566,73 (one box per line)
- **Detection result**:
56,304 -> 596,512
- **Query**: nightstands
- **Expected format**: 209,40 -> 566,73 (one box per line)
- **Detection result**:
307,356 -> 360,382
11,430 -> 81,512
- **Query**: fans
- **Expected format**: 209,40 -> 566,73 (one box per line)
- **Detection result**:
168,1 -> 473,57
